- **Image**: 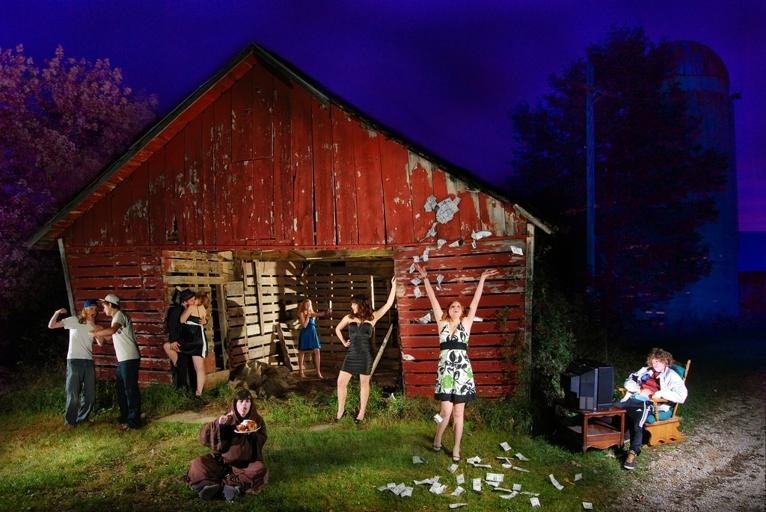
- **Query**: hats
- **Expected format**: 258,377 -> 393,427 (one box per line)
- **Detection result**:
100,294 -> 120,306
179,288 -> 196,302
83,301 -> 97,307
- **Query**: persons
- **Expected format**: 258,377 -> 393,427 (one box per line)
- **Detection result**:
46,296 -> 106,427
333,274 -> 396,423
162,290 -> 209,401
85,292 -> 145,429
185,387 -> 268,501
412,262 -> 503,467
167,288 -> 210,392
607,347 -> 689,472
296,299 -> 333,380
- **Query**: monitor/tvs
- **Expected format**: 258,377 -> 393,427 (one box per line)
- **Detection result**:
560,359 -> 614,412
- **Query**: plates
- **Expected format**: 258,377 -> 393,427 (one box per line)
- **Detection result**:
233,425 -> 262,433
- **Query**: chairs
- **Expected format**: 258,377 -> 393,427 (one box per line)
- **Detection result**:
615,359 -> 692,448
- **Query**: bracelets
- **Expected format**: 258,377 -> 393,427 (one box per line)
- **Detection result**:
422,276 -> 428,279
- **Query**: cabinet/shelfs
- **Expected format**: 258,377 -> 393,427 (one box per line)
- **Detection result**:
549,398 -> 629,456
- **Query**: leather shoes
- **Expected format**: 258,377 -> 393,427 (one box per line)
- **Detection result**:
333,410 -> 365,425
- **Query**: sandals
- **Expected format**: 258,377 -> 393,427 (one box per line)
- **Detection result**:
433,435 -> 443,454
452,452 -> 460,464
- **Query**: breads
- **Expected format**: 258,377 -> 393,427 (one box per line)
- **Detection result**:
225,415 -> 232,427
235,420 -> 257,431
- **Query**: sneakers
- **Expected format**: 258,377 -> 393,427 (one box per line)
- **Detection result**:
223,484 -> 244,503
623,450 -> 638,470
199,484 -> 222,500
